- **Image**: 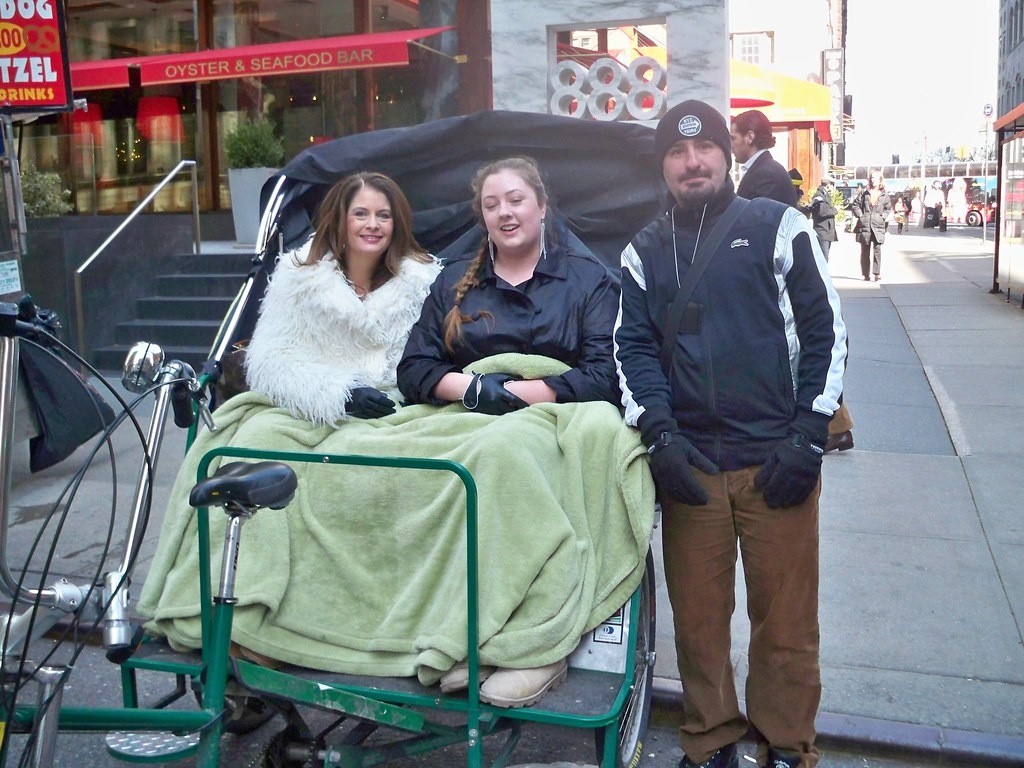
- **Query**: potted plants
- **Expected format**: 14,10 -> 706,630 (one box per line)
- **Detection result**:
220,110 -> 286,245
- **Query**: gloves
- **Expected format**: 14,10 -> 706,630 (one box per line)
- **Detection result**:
458,369 -> 530,416
755,431 -> 823,509
640,418 -> 720,506
344,379 -> 397,420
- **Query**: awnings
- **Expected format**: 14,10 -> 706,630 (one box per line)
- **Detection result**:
140,25 -> 458,87
68,54 -> 180,92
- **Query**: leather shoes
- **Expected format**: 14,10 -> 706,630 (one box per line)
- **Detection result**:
440,656 -> 568,708
824,430 -> 854,452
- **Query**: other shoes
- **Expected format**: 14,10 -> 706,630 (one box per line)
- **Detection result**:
678,738 -> 739,768
875,278 -> 881,281
768,755 -> 798,768
864,278 -> 870,281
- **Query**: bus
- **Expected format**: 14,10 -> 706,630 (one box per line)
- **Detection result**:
822,161 -> 1024,206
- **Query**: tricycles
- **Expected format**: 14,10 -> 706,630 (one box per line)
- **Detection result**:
1,110 -> 668,768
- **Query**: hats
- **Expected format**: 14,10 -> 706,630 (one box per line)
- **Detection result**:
655,99 -> 733,174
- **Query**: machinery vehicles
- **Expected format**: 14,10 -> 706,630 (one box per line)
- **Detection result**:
965,185 -> 997,227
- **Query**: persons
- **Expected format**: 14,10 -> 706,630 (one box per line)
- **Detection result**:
611,99 -> 848,768
852,168 -> 893,282
729,110 -> 853,456
911,180 -> 946,229
787,168 -> 839,264
397,155 -> 622,709
893,194 -> 912,235
219,171 -> 446,672
